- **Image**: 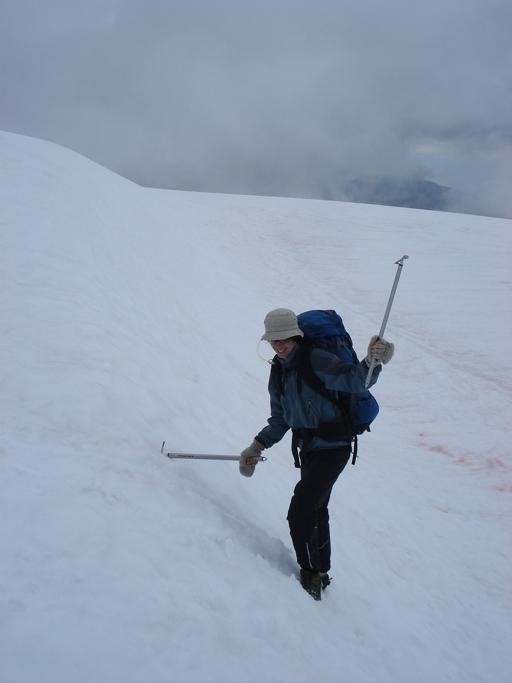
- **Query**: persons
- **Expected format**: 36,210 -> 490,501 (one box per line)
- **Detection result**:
236,307 -> 395,603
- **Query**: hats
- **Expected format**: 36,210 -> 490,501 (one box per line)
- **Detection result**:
260,307 -> 304,342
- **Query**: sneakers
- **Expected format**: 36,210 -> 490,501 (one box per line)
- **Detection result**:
300,570 -> 321,601
318,572 -> 330,590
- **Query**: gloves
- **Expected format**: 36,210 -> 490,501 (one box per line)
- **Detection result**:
367,334 -> 394,368
238,438 -> 265,477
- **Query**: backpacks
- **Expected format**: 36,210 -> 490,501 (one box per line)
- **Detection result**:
277,308 -> 381,438
